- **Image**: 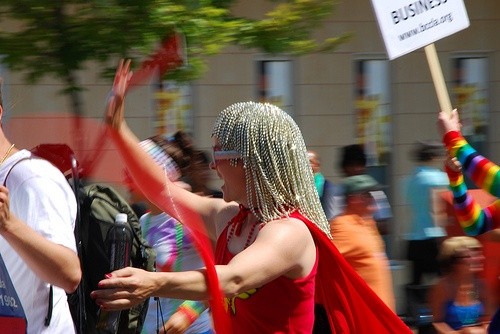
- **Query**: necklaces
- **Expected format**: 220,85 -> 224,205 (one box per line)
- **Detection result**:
1,142 -> 16,167
219,216 -> 262,266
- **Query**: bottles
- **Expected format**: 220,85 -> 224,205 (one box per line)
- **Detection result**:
108,214 -> 132,272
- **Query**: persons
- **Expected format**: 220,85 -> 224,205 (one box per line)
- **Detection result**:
88,58 -> 334,334
438,109 -> 499,334
29,132 -> 486,334
0,89 -> 83,334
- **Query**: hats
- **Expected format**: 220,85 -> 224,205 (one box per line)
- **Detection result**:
437,236 -> 482,257
341,175 -> 389,193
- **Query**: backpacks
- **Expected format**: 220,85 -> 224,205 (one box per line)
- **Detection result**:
66,181 -> 157,333
